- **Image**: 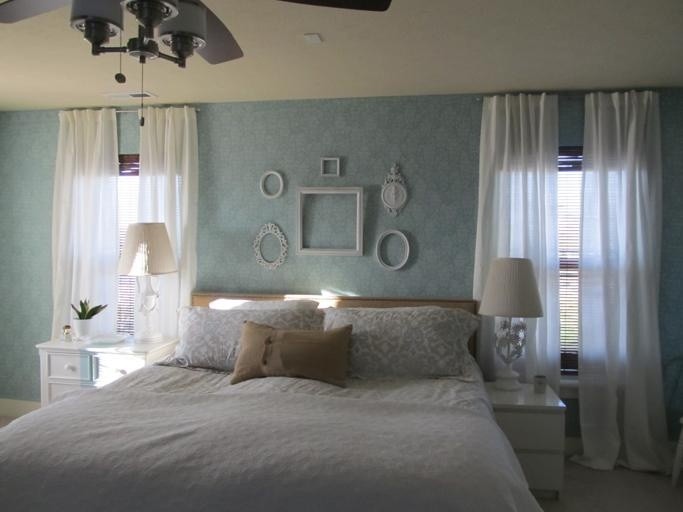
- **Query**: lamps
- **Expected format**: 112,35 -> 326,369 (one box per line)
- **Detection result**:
116,220 -> 180,338
474,256 -> 546,391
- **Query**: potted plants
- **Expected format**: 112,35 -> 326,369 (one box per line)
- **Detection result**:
70,299 -> 107,338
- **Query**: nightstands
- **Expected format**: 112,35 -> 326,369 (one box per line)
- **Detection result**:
487,379 -> 570,499
35,338 -> 180,407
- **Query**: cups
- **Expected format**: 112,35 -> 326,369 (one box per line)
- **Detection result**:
533,374 -> 548,394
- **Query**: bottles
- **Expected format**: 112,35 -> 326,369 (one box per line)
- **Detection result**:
63,325 -> 72,342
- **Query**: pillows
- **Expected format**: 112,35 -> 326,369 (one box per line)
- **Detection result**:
322,303 -> 481,380
159,300 -> 320,369
227,318 -> 353,387
207,296 -> 319,313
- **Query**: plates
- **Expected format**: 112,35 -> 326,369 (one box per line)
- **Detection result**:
88,332 -> 128,346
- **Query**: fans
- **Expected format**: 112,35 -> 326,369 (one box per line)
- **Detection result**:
0,0 -> 393,69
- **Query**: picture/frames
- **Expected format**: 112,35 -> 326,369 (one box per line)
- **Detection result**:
375,230 -> 409,271
320,156 -> 340,179
294,185 -> 363,257
258,171 -> 284,200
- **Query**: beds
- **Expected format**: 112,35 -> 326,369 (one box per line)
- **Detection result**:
0,292 -> 546,512
